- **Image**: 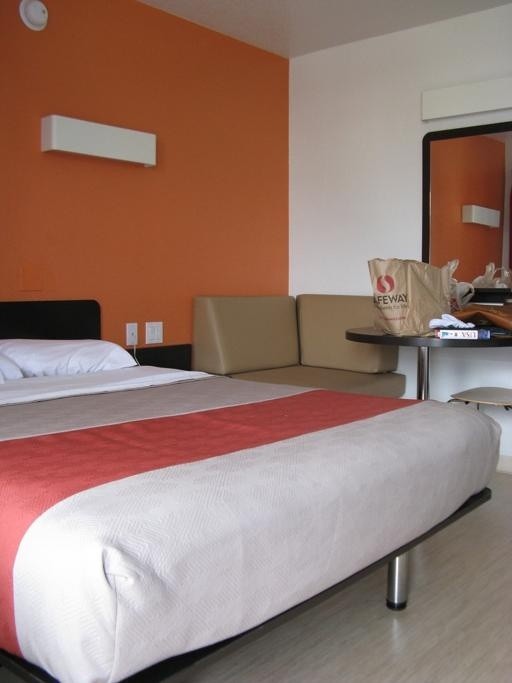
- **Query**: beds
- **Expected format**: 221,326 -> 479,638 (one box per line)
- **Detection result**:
0,298 -> 503,683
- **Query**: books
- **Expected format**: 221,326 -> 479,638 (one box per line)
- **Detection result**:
437,328 -> 492,339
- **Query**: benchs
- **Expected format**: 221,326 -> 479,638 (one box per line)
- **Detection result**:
191,293 -> 405,399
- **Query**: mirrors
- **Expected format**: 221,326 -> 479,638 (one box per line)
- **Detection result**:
421,121 -> 512,304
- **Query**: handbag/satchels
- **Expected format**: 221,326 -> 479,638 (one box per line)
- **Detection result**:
454,303 -> 512,329
368,257 -> 452,337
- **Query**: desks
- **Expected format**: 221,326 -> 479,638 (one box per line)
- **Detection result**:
344,327 -> 512,400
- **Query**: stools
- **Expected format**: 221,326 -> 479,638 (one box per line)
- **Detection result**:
446,387 -> 512,411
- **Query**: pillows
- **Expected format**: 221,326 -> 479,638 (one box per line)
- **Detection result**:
0,338 -> 137,377
0,354 -> 24,382
